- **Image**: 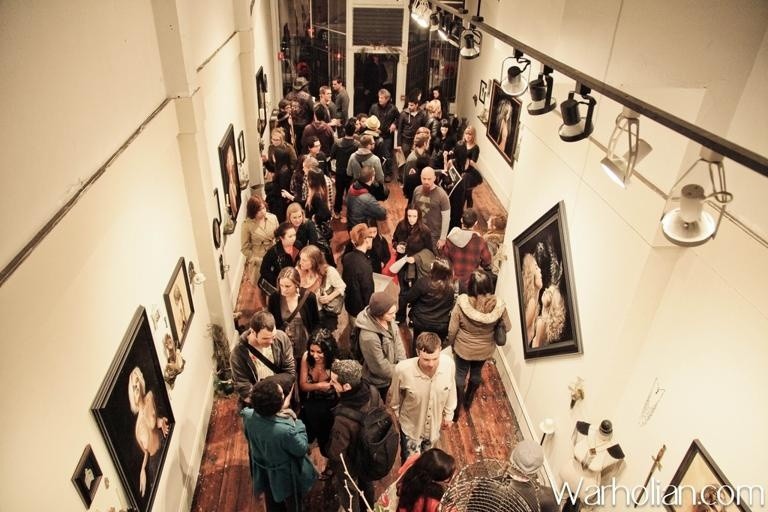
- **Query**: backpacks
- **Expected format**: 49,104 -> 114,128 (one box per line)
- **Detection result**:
332,384 -> 399,480
290,169 -> 303,193
350,327 -> 387,365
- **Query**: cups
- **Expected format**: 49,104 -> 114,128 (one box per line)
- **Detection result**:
397,241 -> 408,253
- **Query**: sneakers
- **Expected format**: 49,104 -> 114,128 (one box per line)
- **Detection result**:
384,175 -> 391,182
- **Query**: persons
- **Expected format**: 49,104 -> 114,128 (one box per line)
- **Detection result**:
127,365 -> 170,499
172,286 -> 188,334
228,53 -> 561,512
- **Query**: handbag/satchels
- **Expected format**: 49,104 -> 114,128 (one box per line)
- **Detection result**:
303,481 -> 341,512
440,171 -> 452,185
373,182 -> 389,197
463,165 -> 482,189
404,264 -> 415,282
494,319 -> 506,345
373,482 -> 399,512
322,286 -> 343,315
320,220 -> 333,240
258,276 -> 277,297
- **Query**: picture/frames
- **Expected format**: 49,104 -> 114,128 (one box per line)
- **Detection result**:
511,200 -> 584,360
70,257 -> 196,512
479,78 -> 523,170
661,439 -> 751,512
256,65 -> 268,136
216,123 -> 246,220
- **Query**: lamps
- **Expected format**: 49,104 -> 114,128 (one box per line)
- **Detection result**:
407,0 -> 484,60
500,48 -> 531,98
527,64 -> 557,116
660,145 -> 734,248
559,80 -> 597,143
600,104 -> 653,188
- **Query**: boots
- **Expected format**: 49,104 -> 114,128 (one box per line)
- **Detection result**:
453,385 -> 465,422
462,378 -> 479,410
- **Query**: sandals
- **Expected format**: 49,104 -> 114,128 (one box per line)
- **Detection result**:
319,460 -> 333,480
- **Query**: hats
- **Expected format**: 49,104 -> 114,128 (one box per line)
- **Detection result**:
365,115 -> 381,130
349,223 -> 377,248
369,292 -> 395,316
510,440 -> 544,475
293,76 -> 310,90
278,111 -> 289,122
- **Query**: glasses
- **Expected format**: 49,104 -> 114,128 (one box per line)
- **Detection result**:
271,138 -> 280,142
